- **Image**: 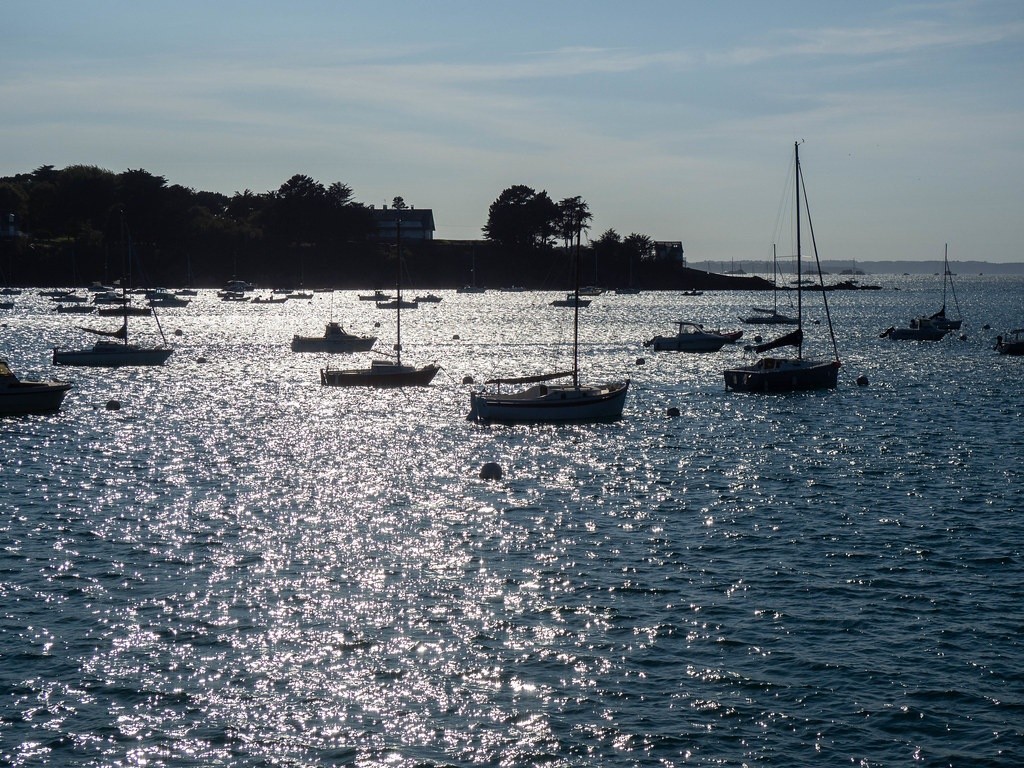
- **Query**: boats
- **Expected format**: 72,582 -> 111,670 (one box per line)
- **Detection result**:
0,281 -> 198,316
412,285 -> 525,303
644,321 -> 729,351
0,356 -> 73,416
943,267 -> 957,275
291,322 -> 378,352
879,326 -> 951,340
991,328 -> 1024,356
358,290 -> 391,300
705,325 -> 744,343
376,300 -> 418,309
217,275 -> 334,303
549,294 -> 592,307
577,286 -> 641,296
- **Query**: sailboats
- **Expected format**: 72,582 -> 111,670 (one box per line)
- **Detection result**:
320,218 -> 440,387
737,242 -> 798,324
909,242 -> 964,330
53,207 -> 175,366
722,140 -> 842,395
